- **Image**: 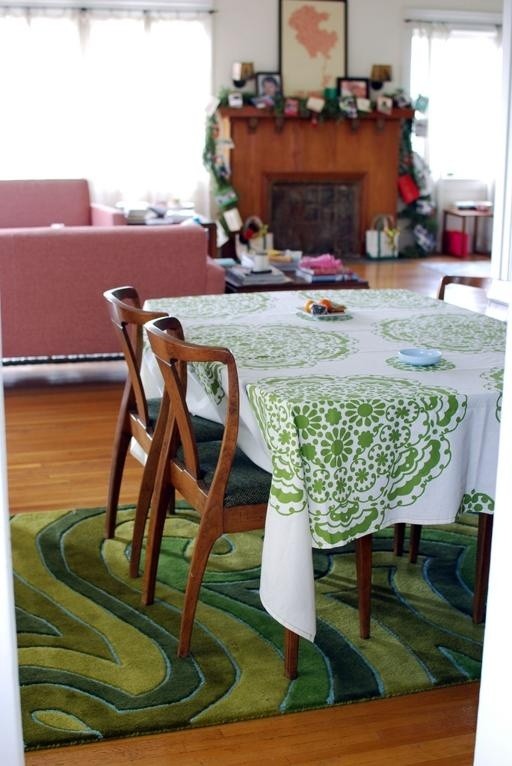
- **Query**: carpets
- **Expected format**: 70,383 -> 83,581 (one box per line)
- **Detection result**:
7,506 -> 487,757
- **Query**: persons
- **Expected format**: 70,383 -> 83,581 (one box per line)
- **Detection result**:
260,76 -> 281,99
349,82 -> 365,96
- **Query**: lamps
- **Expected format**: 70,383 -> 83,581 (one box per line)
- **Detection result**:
233,60 -> 256,87
371,61 -> 393,90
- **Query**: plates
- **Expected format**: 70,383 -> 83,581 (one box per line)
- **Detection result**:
397,347 -> 442,366
296,305 -> 349,321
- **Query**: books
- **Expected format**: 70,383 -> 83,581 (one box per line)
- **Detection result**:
295,269 -> 360,283
212,250 -> 298,285
117,201 -> 198,227
297,263 -> 353,275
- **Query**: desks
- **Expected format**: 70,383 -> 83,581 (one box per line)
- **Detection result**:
146,287 -> 507,628
439,209 -> 493,256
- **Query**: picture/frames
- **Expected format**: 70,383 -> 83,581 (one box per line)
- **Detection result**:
335,77 -> 369,97
278,0 -> 350,97
253,71 -> 282,101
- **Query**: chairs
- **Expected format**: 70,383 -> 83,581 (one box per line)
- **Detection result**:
102,287 -> 226,580
142,317 -> 374,660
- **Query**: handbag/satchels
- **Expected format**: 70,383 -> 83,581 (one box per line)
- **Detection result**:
365,214 -> 401,261
235,215 -> 274,262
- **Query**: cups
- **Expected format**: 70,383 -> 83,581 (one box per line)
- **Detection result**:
253,252 -> 270,271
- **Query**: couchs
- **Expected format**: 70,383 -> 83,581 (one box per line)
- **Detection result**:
0,174 -> 226,367
